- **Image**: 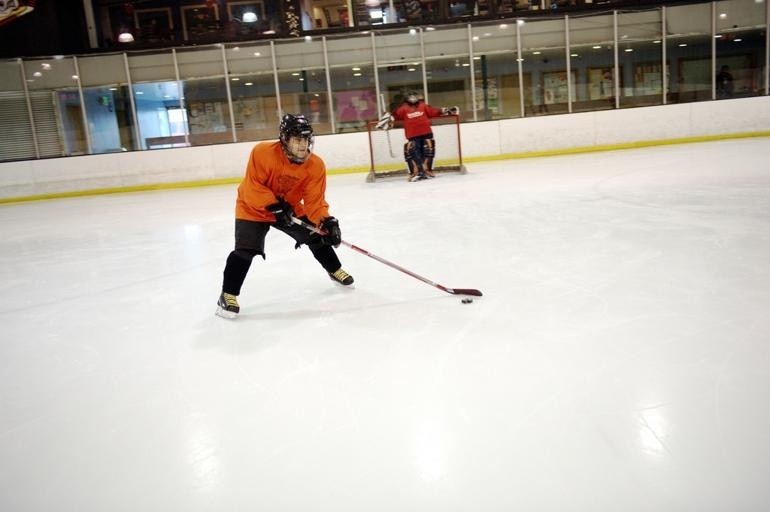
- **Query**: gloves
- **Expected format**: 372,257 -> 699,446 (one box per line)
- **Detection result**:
440,105 -> 460,117
264,195 -> 296,230
318,215 -> 342,248
375,112 -> 396,131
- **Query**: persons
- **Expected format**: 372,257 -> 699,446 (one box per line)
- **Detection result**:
716,65 -> 733,100
378,90 -> 459,182
217,112 -> 355,314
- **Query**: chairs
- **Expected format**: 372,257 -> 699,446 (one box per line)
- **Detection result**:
321,7 -> 342,28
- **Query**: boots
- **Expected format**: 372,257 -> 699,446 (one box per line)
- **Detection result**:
216,290 -> 240,314
328,267 -> 355,286
403,141 -> 424,183
422,138 -> 436,179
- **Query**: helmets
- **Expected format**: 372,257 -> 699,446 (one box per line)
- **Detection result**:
278,112 -> 315,164
405,90 -> 419,109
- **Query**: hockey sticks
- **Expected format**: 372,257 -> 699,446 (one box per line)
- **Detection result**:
381,94 -> 398,157
292,217 -> 482,296
